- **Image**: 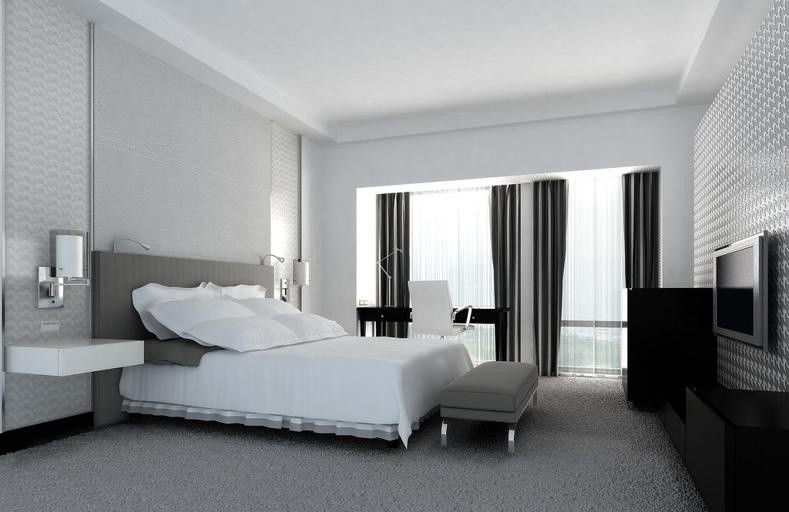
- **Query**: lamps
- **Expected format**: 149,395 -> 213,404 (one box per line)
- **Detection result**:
373,248 -> 406,308
110,237 -> 153,256
35,226 -> 92,312
261,252 -> 284,266
279,257 -> 311,305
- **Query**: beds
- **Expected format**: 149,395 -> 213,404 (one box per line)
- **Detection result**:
88,245 -> 478,450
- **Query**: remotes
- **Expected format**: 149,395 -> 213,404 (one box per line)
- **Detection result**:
715,245 -> 728,251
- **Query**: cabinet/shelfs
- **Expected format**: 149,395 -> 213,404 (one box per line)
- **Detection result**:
618,287 -> 720,411
656,374 -> 789,512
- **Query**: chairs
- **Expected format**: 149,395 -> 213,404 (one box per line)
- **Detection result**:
406,279 -> 475,342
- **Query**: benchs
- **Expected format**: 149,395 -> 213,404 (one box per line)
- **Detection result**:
438,355 -> 539,456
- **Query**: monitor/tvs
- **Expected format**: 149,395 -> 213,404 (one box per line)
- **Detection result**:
713,230 -> 768,352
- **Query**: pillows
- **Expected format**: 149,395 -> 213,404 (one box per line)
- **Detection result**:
125,279 -> 350,356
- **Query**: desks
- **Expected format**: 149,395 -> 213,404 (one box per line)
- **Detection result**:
357,303 -> 512,364
